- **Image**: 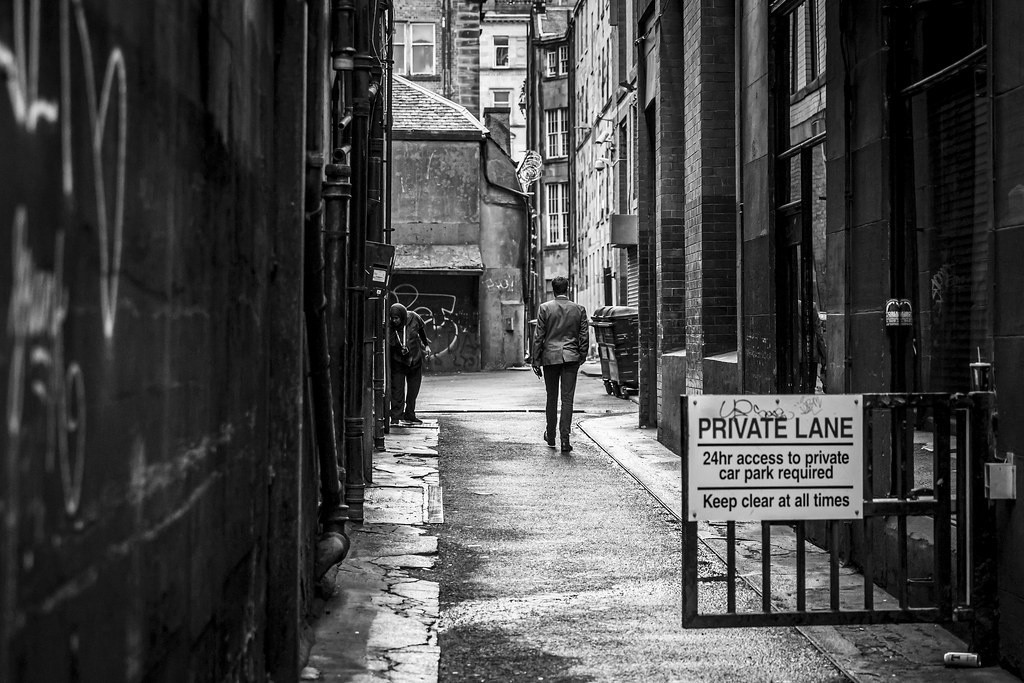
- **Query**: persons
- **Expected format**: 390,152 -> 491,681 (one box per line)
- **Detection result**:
530,276 -> 590,452
389,303 -> 432,427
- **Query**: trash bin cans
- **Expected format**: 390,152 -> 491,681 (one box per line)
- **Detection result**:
528,319 -> 538,364
591,305 -> 639,396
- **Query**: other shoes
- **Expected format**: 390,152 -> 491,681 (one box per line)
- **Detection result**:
544,431 -> 556,447
561,440 -> 574,451
402,416 -> 423,424
389,418 -> 400,426
